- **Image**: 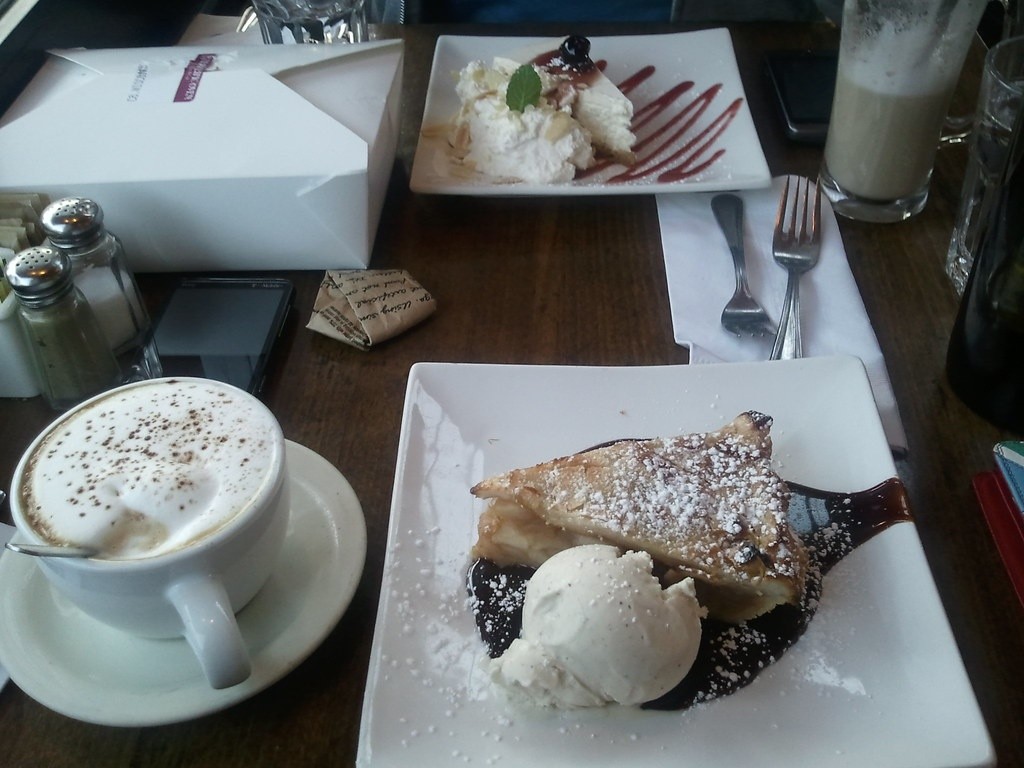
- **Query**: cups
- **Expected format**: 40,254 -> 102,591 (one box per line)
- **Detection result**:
818,0 -> 992,221
252,0 -> 368,44
947,96 -> 1024,441
945,37 -> 1024,296
10,377 -> 289,690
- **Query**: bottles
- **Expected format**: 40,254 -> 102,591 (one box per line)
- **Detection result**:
7,247 -> 122,413
41,196 -> 163,386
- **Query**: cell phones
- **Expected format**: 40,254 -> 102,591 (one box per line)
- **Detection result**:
765,48 -> 838,142
123,276 -> 297,398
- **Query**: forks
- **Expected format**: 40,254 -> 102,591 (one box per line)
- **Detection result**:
768,174 -> 822,359
711,194 -> 778,337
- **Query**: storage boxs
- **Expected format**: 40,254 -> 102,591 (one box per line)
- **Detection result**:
0,38 -> 404,272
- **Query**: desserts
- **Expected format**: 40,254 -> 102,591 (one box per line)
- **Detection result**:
465,412 -> 810,711
422,32 -> 637,186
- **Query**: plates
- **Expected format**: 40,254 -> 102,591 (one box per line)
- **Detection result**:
0,439 -> 367,730
357,355 -> 995,768
408,28 -> 772,194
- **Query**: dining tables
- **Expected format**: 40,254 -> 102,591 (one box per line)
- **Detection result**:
0,22 -> 1024,768
